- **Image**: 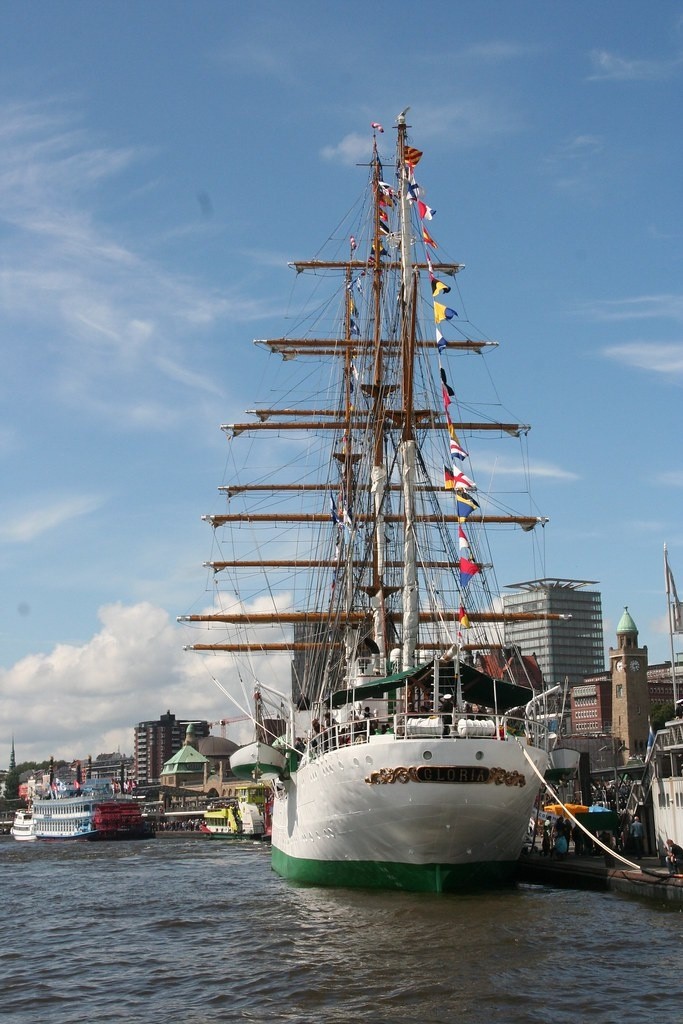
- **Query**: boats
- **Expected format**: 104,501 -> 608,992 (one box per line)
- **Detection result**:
228,739 -> 287,784
200,799 -> 267,839
10,807 -> 38,842
544,748 -> 580,782
32,756 -> 156,841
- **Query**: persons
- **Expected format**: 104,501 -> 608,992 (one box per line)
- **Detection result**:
441,694 -> 453,735
140,800 -> 236,832
325,712 -> 341,751
296,737 -> 305,762
572,823 -> 584,855
359,707 -> 378,735
313,719 -> 329,751
297,693 -> 310,710
542,816 -> 573,858
467,703 -> 486,720
630,816 -> 645,860
348,710 -> 362,730
664,839 -> 683,874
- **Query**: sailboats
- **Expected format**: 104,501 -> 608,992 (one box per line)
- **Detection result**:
174,107 -> 572,895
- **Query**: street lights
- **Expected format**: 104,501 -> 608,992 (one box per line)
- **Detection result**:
599,737 -> 631,817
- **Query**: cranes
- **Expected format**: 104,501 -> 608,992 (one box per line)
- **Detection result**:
207,710 -> 274,739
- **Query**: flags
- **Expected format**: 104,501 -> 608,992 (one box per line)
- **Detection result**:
404,144 -> 424,168
455,490 -> 482,629
407,176 -> 459,323
327,236 -> 356,554
436,328 -> 477,489
